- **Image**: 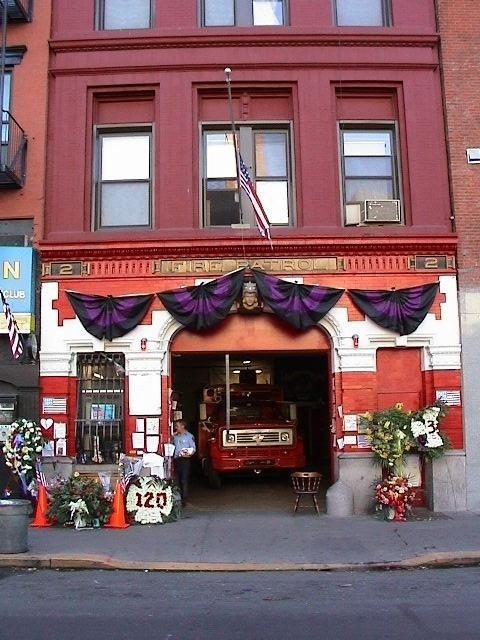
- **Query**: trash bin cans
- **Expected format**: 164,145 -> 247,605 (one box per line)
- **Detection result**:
1,499 -> 32,553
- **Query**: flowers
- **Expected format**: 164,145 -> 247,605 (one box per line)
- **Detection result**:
354,398 -> 455,522
3,417 -> 180,529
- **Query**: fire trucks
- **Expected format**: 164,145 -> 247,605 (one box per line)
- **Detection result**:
194,383 -> 308,489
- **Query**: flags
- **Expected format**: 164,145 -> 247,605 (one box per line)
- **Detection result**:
237,153 -> 273,241
2,297 -> 24,359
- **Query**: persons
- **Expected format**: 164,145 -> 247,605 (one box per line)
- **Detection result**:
171,418 -> 196,508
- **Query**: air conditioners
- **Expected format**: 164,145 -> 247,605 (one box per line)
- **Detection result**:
360,198 -> 401,223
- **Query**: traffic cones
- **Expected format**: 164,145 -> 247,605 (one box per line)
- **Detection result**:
28,486 -> 58,529
104,485 -> 129,527
